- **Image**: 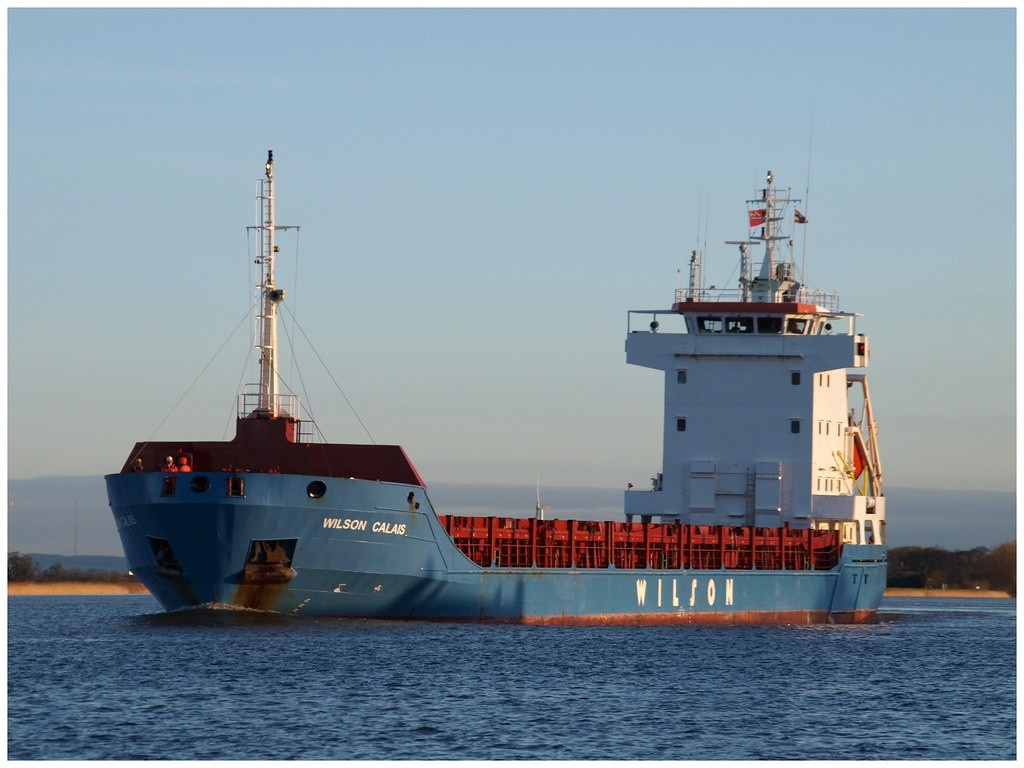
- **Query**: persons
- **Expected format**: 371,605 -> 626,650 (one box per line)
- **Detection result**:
130,456 -> 192,473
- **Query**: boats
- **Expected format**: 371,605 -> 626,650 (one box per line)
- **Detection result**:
101,148 -> 891,627
